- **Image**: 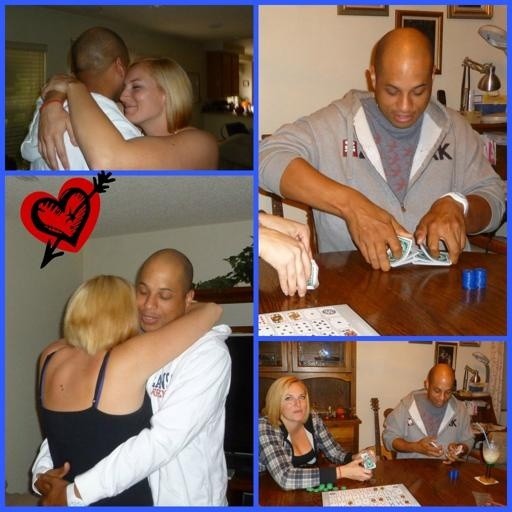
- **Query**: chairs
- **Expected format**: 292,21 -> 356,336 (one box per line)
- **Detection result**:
257,135 -> 319,251
372,398 -> 401,461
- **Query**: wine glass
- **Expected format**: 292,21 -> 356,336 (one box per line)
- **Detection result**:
479,440 -> 503,484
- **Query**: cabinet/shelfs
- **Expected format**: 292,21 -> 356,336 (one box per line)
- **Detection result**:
258,342 -> 361,461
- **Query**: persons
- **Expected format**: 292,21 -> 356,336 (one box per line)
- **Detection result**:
258,28 -> 507,271
258,375 -> 377,491
20,27 -> 219,170
382,364 -> 475,465
258,211 -> 313,297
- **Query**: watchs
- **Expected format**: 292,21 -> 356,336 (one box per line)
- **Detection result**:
440,191 -> 469,215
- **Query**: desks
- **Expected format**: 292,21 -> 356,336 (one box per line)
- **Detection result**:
259,459 -> 508,508
259,252 -> 507,337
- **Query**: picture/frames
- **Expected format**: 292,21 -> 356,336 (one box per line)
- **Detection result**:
433,341 -> 458,373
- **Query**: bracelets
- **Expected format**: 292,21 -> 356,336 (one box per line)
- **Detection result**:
39,97 -> 63,113
258,209 -> 267,213
338,466 -> 341,479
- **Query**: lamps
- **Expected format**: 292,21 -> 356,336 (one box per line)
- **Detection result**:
458,363 -> 480,394
